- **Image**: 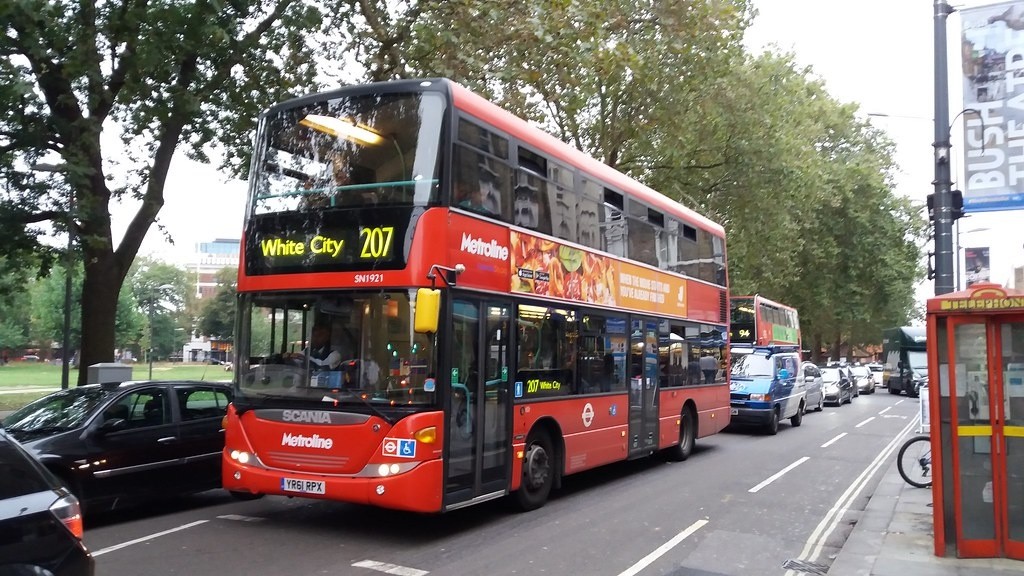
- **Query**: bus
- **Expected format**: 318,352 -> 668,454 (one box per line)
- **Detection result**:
730,296 -> 801,360
222,75 -> 730,515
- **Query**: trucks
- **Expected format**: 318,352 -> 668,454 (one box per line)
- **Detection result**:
883,327 -> 929,398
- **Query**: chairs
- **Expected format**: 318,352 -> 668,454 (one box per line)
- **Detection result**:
134,400 -> 162,428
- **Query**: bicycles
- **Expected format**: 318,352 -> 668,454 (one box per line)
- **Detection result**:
897,436 -> 931,488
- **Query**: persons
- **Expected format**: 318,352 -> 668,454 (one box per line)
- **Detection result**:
456,178 -> 499,216
283,322 -> 341,372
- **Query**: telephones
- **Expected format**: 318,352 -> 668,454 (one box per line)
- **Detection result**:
968,370 -> 1012,421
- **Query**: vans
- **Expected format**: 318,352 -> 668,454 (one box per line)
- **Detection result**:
730,343 -> 807,435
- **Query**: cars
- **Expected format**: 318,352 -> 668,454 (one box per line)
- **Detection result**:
0,428 -> 95,576
0,382 -> 265,522
817,363 -> 894,406
801,361 -> 825,414
